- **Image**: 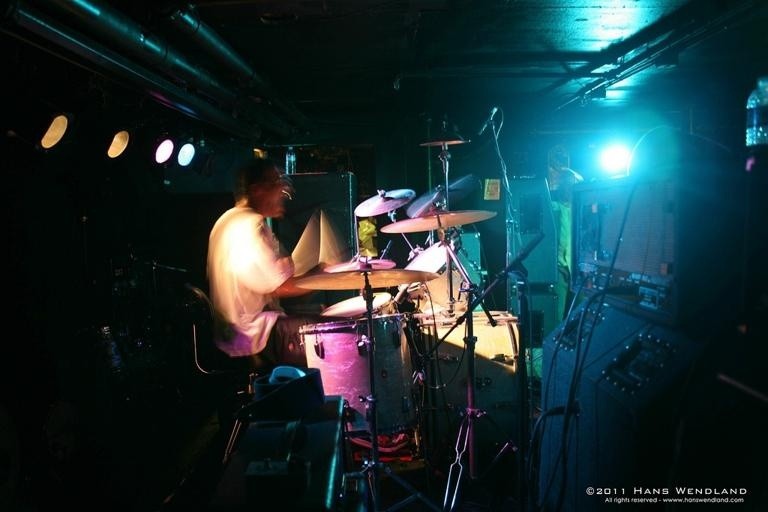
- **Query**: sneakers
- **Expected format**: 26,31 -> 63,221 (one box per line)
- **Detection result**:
352,448 -> 415,462
347,430 -> 409,453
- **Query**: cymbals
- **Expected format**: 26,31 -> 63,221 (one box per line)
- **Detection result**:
324,259 -> 396,273
406,171 -> 482,219
292,269 -> 440,291
419,139 -> 470,145
354,187 -> 416,218
380,210 -> 498,234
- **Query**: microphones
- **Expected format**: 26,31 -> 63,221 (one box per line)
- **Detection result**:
469,106 -> 500,142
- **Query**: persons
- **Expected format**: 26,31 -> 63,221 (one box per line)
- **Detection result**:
201,161 -> 411,454
548,167 -> 583,328
358,216 -> 378,257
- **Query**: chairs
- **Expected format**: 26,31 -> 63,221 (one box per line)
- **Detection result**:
184,270 -> 298,468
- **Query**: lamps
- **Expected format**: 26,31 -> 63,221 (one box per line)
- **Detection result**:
39,113 -> 209,174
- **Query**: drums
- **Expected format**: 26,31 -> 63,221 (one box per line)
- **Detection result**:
315,293 -> 399,318
398,240 -> 475,313
405,309 -> 530,482
298,314 -> 419,436
